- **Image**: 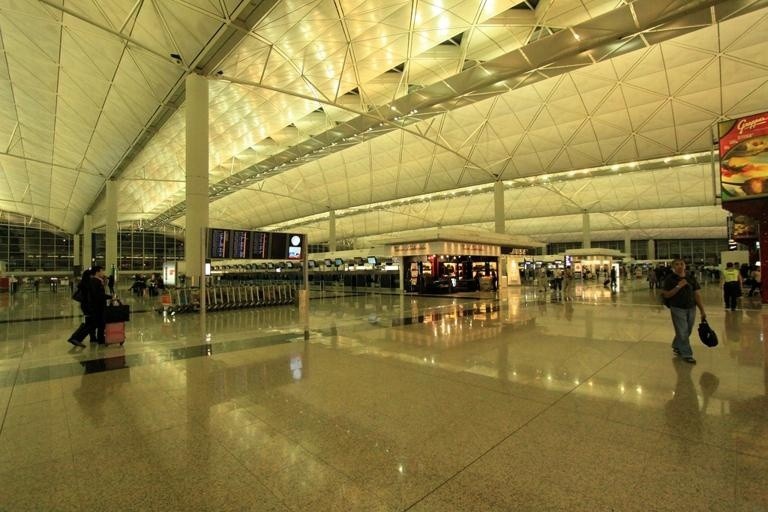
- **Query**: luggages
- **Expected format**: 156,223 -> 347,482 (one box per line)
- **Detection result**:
103,321 -> 125,347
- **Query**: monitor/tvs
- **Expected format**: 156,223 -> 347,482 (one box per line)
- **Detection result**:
524,260 -> 565,269
211,256 -> 376,271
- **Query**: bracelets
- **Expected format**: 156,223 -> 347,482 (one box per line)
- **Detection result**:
675,285 -> 681,290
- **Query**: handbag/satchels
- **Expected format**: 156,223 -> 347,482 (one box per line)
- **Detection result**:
104,298 -> 130,324
698,319 -> 719,348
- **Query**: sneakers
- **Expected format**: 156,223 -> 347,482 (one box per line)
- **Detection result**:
673,347 -> 695,363
67,339 -> 86,347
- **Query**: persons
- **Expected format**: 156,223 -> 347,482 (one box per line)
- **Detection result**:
660,259 -> 707,364
537,264 -> 617,293
67,266 -> 112,348
646,258 -> 761,311
135,272 -> 158,297
